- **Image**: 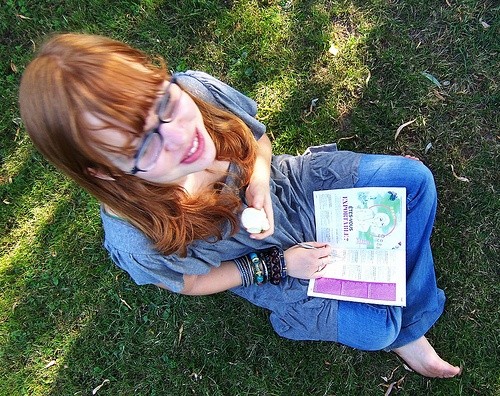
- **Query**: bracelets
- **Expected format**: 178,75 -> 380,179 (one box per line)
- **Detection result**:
232,246 -> 287,287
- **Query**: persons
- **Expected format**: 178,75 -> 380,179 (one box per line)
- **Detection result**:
18,33 -> 462,380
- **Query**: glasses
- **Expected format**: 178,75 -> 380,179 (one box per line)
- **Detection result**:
106,74 -> 182,178
